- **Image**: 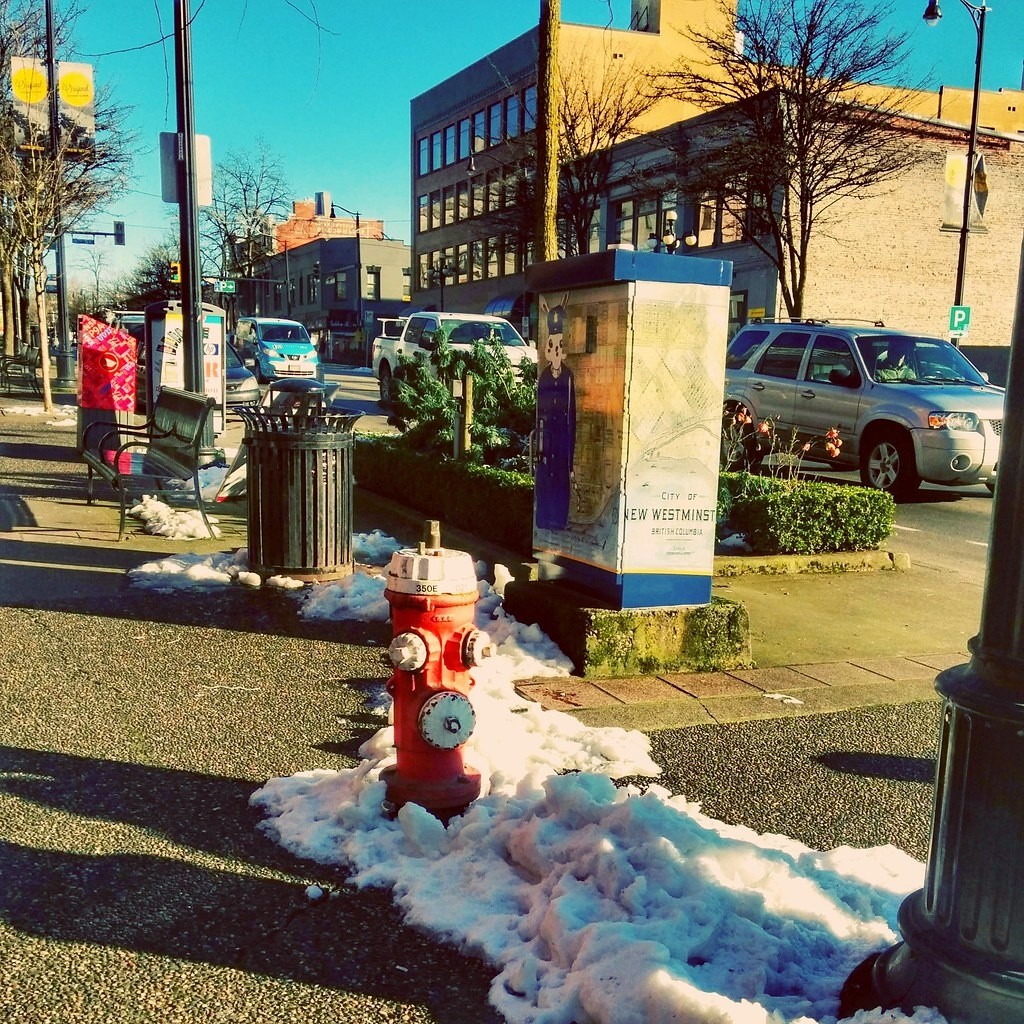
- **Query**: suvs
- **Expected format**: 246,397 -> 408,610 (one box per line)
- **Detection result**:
131,331 -> 261,416
721,315 -> 1006,501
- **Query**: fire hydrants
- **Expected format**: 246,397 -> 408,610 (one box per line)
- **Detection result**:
380,519 -> 497,830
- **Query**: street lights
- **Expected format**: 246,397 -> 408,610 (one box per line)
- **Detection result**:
465,150 -> 530,271
647,210 -> 697,256
329,201 -> 364,330
261,235 -> 291,316
924,0 -> 988,349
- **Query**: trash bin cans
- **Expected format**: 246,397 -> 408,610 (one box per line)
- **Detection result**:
231,376 -> 367,587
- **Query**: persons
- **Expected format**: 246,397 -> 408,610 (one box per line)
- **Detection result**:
477,326 -> 501,344
874,338 -> 917,383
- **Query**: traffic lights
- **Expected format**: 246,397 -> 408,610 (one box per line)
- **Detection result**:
312,263 -> 320,280
170,263 -> 181,283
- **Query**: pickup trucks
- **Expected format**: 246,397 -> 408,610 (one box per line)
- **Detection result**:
371,312 -> 539,412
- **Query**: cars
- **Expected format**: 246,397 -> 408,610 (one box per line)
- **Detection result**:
107,311 -> 147,340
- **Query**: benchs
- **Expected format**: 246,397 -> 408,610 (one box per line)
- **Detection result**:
0,343 -> 44,398
82,385 -> 219,542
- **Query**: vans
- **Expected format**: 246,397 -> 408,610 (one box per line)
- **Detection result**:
234,317 -> 317,380
